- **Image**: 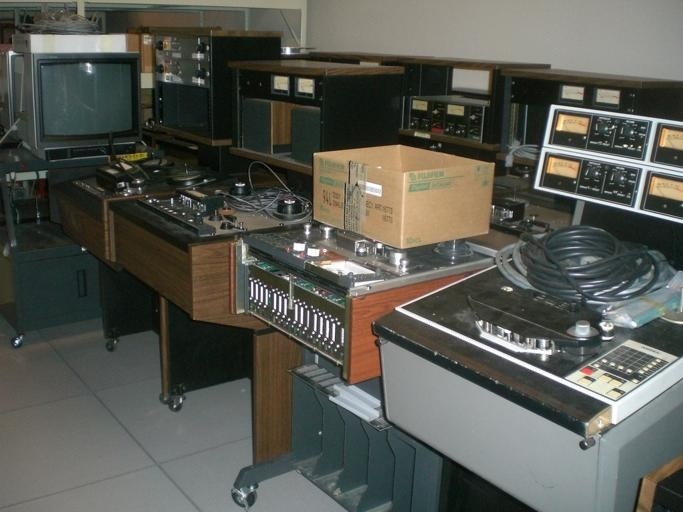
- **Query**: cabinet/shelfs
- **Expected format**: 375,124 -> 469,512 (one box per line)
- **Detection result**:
0,147 -> 100,332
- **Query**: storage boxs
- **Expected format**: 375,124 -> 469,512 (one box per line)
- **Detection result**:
313,142 -> 498,250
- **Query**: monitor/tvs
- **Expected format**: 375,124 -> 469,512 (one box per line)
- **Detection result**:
24,53 -> 142,151
